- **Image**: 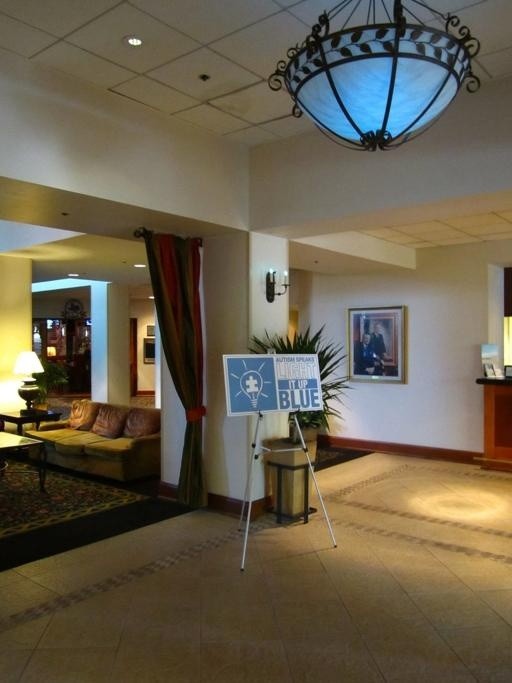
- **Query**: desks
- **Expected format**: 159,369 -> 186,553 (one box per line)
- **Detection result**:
0,410 -> 59,435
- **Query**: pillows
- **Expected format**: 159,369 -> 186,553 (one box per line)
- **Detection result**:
68,400 -> 160,439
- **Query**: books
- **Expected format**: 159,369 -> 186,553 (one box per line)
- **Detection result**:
480,343 -> 498,377
484,363 -> 496,378
492,363 -> 505,378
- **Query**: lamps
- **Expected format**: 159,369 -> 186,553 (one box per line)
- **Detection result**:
15,351 -> 45,416
266,272 -> 291,303
268,0 -> 480,152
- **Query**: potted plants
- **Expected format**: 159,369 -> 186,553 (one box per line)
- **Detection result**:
34,358 -> 69,411
249,324 -> 353,455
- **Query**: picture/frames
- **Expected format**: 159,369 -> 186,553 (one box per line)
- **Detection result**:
347,305 -> 407,385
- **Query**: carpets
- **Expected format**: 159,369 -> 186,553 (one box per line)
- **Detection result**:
0,460 -> 196,573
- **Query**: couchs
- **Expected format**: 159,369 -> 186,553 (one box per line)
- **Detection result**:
25,403 -> 160,483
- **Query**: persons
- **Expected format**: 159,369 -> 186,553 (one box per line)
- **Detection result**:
368,323 -> 388,360
353,333 -> 385,373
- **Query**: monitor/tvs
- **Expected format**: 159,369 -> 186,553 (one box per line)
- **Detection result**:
46,318 -> 60,332
143,338 -> 156,364
85,318 -> 91,326
